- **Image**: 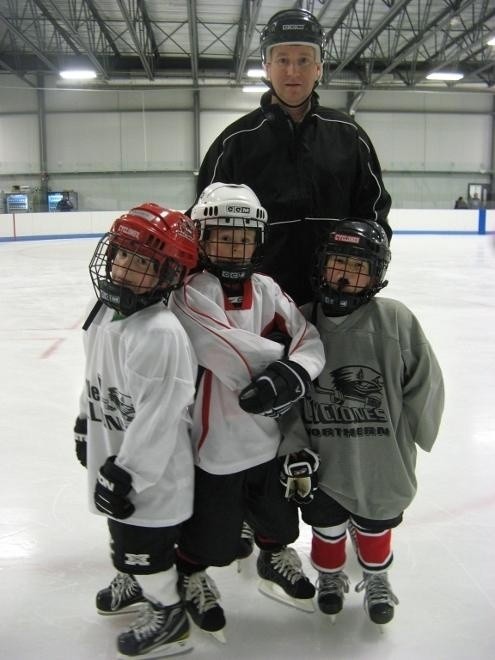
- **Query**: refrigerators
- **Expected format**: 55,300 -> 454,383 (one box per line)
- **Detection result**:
5,191 -> 34,213
48,191 -> 79,211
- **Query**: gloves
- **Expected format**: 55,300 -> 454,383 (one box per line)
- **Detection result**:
279,449 -> 319,505
94,455 -> 134,520
239,358 -> 312,420
73,416 -> 87,469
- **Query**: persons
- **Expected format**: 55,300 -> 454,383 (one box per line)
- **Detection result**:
470,193 -> 481,209
74,204 -> 195,659
276,216 -> 446,626
179,8 -> 391,562
166,181 -> 326,635
455,196 -> 468,209
55,193 -> 73,212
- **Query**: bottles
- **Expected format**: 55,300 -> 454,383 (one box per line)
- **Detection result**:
9,199 -> 26,209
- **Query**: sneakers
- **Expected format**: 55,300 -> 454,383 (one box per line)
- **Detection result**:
257,545 -> 316,612
116,600 -> 194,660
236,524 -> 254,573
355,571 -> 397,633
315,571 -> 351,623
97,572 -> 149,614
176,569 -> 226,643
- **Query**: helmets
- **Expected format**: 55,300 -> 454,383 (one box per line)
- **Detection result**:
259,8 -> 328,66
307,216 -> 391,316
190,182 -> 269,285
88,203 -> 199,316
